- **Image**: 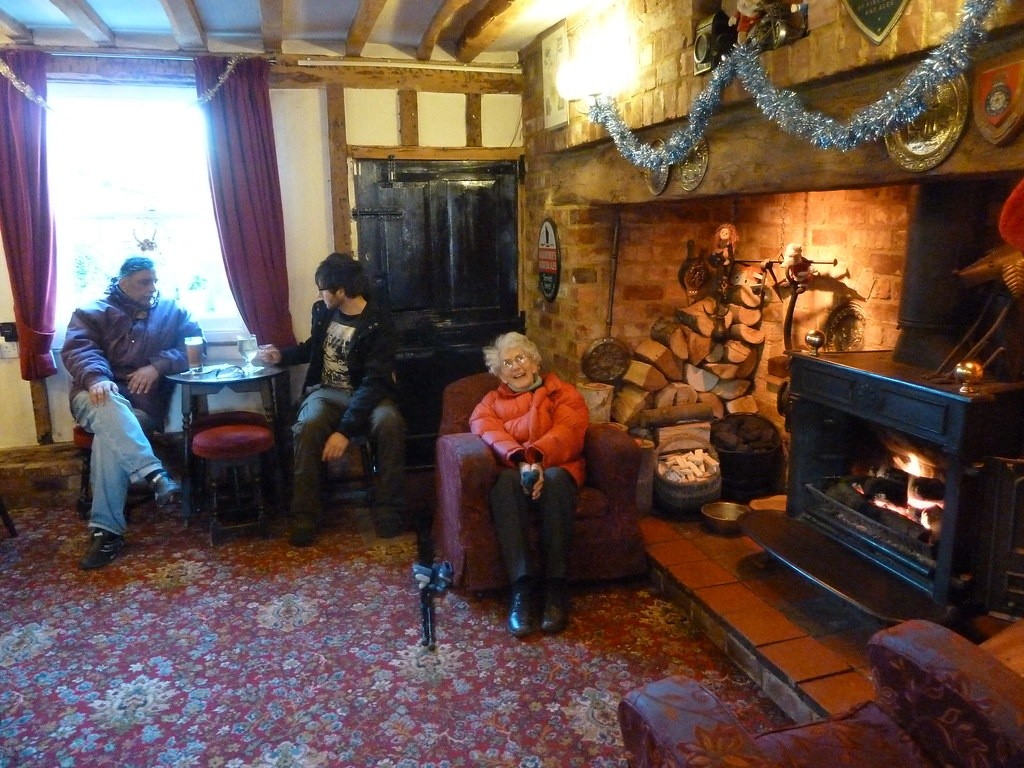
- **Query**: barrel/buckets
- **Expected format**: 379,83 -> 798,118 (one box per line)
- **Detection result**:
712,411 -> 784,504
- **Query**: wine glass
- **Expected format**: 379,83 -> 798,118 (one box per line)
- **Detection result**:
236,335 -> 259,374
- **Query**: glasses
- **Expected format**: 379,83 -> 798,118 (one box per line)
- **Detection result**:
501,353 -> 528,369
318,287 -> 328,293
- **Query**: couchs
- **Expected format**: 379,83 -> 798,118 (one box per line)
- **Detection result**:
618,620 -> 1024,768
426,374 -> 641,596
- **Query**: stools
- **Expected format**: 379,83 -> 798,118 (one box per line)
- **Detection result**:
73,427 -> 157,522
321,427 -> 381,508
192,410 -> 276,549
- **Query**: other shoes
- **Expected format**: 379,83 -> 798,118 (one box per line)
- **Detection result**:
292,528 -> 314,545
376,516 -> 403,537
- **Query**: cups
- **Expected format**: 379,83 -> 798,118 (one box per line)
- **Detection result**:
185,336 -> 203,374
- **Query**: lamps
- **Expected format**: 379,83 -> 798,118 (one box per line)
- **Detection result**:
555,55 -> 616,115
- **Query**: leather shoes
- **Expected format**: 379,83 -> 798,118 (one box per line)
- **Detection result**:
539,579 -> 569,631
507,583 -> 534,635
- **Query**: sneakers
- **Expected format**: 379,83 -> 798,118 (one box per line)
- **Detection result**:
79,529 -> 125,568
147,468 -> 184,505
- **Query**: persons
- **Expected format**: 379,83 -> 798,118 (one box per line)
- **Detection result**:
468,332 -> 589,636
60,257 -> 208,567
259,252 -> 407,547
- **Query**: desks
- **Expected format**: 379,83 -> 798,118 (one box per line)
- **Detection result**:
164,362 -> 292,528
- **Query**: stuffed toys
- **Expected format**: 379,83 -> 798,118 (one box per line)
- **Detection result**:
711,223 -> 739,280
780,243 -> 818,294
730,262 -> 763,287
728,0 -> 764,45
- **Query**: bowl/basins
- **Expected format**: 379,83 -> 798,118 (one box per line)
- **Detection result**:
701,502 -> 749,531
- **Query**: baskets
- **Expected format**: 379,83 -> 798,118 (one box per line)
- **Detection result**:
654,433 -> 721,508
